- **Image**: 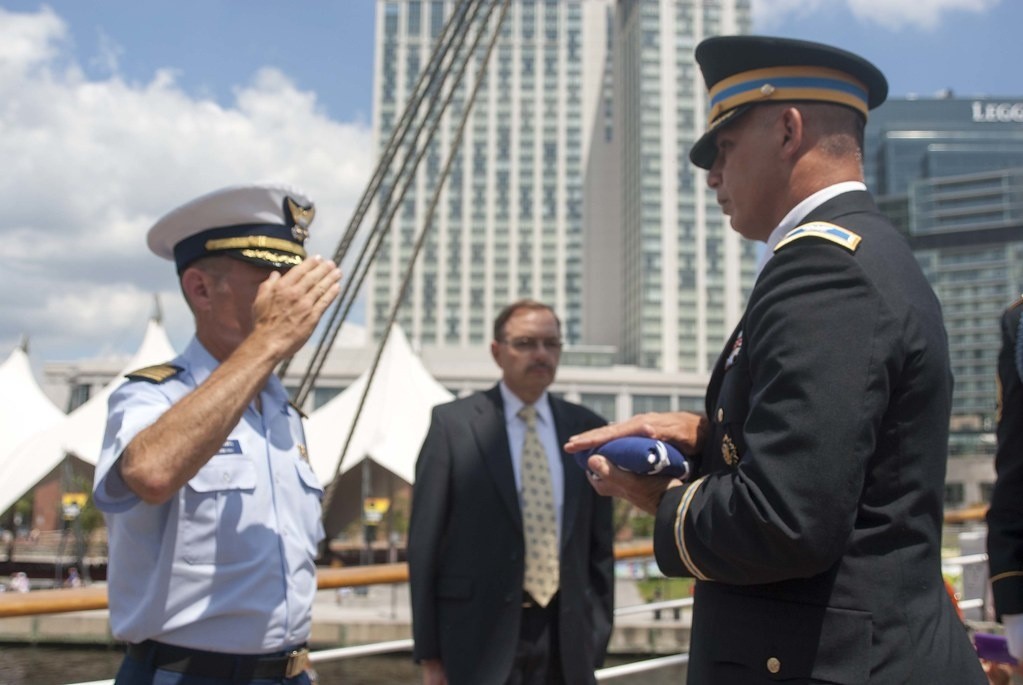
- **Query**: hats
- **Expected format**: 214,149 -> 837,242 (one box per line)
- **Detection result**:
147,185 -> 315,269
689,35 -> 888,171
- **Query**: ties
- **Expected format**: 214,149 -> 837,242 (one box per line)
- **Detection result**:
517,406 -> 562,608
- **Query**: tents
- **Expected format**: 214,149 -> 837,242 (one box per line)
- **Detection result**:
307,328 -> 458,497
66,311 -> 205,469
0,338 -> 67,535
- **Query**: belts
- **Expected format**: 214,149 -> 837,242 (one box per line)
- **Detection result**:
521,592 -> 560,611
125,638 -> 309,678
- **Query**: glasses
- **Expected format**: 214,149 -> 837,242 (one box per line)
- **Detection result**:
499,334 -> 562,353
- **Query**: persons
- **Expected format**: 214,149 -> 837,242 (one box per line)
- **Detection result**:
90,183 -> 345,685
560,34 -> 998,684
985,301 -> 1022,669
409,300 -> 623,685
